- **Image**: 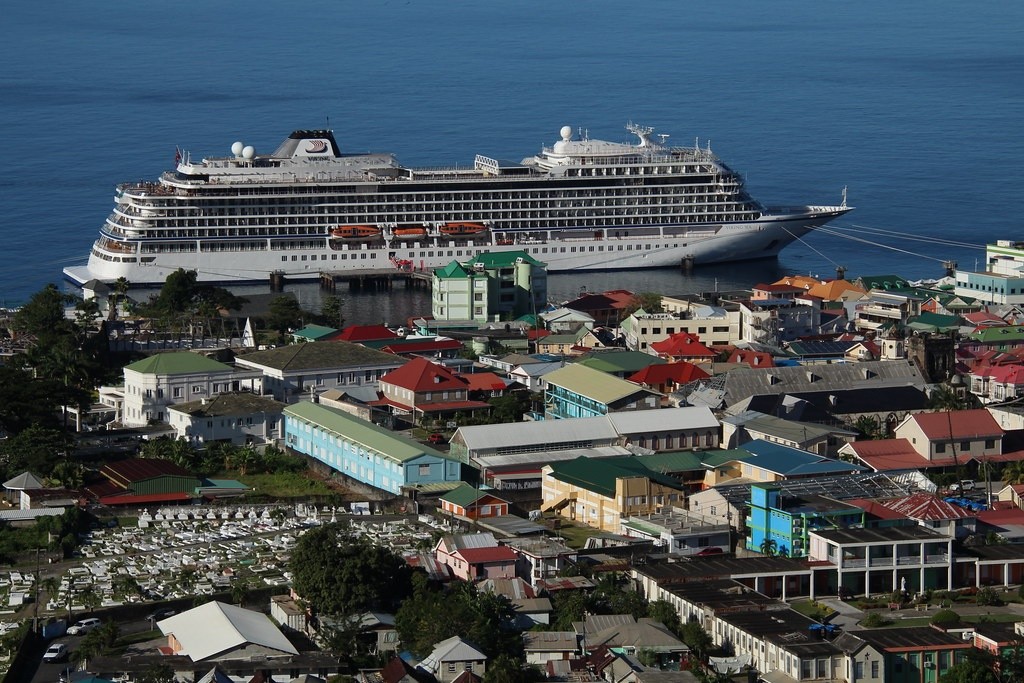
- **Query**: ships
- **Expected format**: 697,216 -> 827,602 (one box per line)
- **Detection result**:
59,117 -> 857,292
